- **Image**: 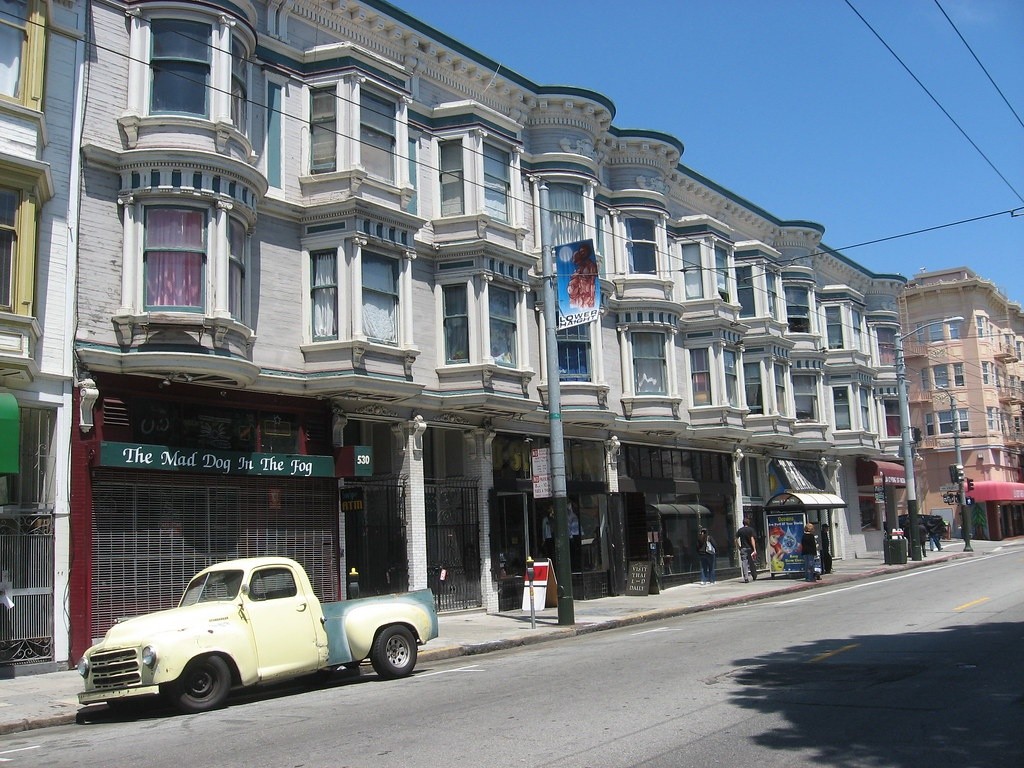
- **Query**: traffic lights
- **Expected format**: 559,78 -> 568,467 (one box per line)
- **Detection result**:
966,477 -> 974,491
949,464 -> 964,484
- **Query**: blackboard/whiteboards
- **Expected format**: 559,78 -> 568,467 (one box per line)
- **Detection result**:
625,559 -> 660,596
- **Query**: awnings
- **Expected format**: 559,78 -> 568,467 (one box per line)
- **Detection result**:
0,393 -> 19,474
967,482 -> 1024,502
856,457 -> 906,486
777,459 -> 836,494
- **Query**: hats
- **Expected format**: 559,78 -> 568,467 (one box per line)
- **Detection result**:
822,524 -> 830,527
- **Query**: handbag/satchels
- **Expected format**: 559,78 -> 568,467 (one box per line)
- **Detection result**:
705,535 -> 715,554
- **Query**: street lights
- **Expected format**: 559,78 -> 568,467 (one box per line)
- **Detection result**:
895,316 -> 964,560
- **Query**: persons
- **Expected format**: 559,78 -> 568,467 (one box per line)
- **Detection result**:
820,524 -> 832,574
735,519 -> 757,583
904,517 -> 943,557
801,523 -> 822,582
697,528 -> 719,585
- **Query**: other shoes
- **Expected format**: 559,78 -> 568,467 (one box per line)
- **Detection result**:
753,575 -> 757,580
711,581 -> 716,585
701,582 -> 706,585
924,556 -> 927,557
938,548 -> 943,551
745,579 -> 749,583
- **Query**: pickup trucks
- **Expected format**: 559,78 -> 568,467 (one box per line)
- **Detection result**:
77,555 -> 439,714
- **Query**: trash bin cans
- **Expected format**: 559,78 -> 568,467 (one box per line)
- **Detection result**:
883,533 -> 908,565
